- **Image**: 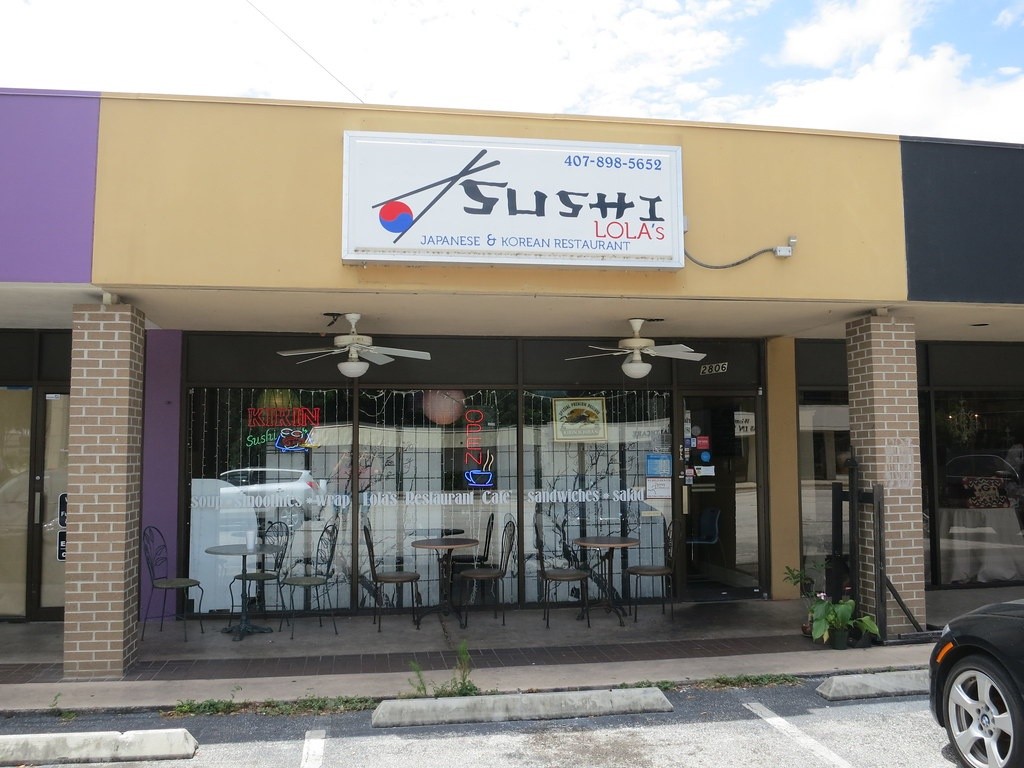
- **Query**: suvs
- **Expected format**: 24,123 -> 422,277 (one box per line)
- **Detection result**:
211,467 -> 329,533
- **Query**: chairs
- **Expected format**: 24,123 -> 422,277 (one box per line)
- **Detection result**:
534,523 -> 592,629
627,520 -> 681,624
282,525 -> 338,639
229,521 -> 290,633
504,514 -> 545,604
687,508 -> 726,567
141,526 -> 204,642
446,513 -> 494,609
363,525 -> 421,631
460,521 -> 515,626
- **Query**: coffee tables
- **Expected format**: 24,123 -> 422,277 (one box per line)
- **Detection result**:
574,537 -> 640,626
205,544 -> 282,641
411,538 -> 479,628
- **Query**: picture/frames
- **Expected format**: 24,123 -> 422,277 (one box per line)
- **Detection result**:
552,397 -> 608,442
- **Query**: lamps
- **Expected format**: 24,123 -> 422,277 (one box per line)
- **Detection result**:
622,354 -> 651,378
337,356 -> 369,378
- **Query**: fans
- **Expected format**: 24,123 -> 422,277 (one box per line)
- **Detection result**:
565,319 -> 706,361
277,313 -> 430,365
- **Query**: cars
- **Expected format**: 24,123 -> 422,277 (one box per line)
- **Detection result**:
935,450 -> 1023,503
928,597 -> 1024,768
